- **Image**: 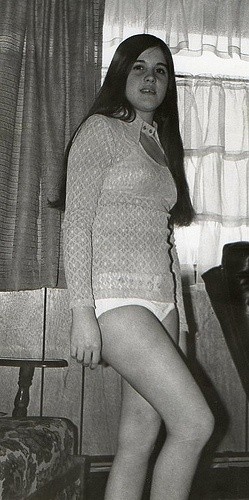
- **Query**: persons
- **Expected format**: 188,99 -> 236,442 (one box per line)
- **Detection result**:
46,33 -> 216,500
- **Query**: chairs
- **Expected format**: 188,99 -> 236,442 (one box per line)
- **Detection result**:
200,241 -> 249,398
0,356 -> 90,499
